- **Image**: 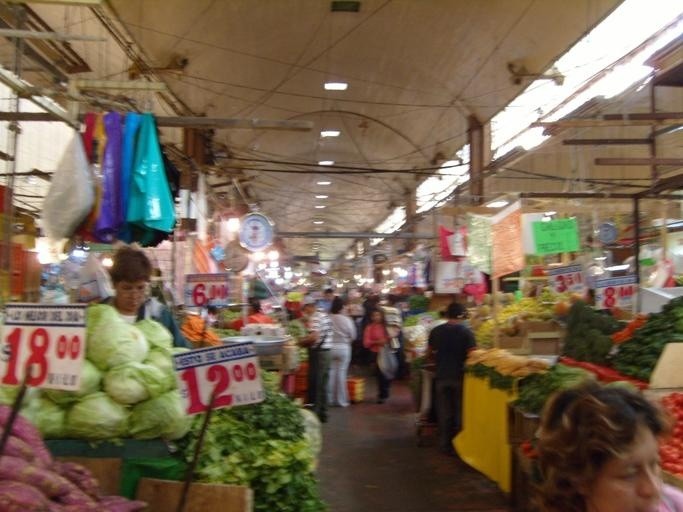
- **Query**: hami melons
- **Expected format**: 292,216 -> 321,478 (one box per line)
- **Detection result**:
479,292 -> 513,317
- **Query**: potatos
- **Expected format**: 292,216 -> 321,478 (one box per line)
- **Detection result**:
0,406 -> 152,512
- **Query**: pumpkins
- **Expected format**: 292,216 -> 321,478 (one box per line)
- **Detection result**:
555,301 -> 569,317
569,293 -> 582,304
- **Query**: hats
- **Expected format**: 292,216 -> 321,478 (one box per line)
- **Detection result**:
439,302 -> 469,320
300,297 -> 316,304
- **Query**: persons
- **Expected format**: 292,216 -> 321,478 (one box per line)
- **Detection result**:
296,283 -> 411,423
518,378 -> 683,512
421,301 -> 480,455
102,243 -> 193,348
214,296 -> 277,331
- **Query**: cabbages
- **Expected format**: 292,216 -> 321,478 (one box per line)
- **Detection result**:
0,304 -> 195,441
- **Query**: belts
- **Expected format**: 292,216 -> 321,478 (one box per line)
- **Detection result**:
308,349 -> 332,352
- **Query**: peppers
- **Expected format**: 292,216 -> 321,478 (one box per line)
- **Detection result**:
610,296 -> 682,380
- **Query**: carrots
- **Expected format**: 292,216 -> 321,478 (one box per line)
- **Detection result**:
179,313 -> 220,345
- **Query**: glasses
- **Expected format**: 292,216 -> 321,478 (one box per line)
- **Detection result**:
116,287 -> 149,294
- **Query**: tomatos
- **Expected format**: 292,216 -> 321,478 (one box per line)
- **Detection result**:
654,394 -> 683,477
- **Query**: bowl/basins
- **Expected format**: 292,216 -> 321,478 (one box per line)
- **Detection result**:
223,335 -> 288,357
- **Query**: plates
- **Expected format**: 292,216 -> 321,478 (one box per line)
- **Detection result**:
239,212 -> 273,252
